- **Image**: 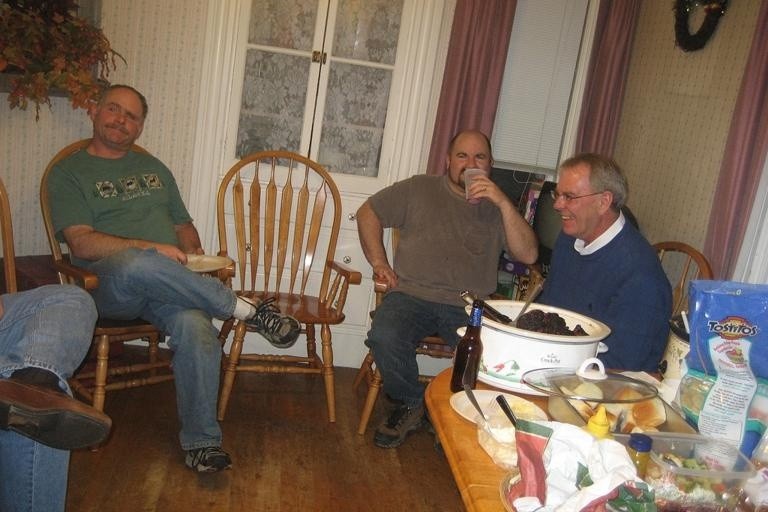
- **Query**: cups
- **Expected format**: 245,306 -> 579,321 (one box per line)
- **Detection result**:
464,169 -> 487,205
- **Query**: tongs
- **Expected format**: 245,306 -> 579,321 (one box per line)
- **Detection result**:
460,292 -> 513,327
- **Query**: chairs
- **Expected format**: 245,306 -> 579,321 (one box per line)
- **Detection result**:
0,177 -> 16,292
353,273 -> 455,435
213,151 -> 362,423
651,241 -> 713,319
40,139 -> 227,452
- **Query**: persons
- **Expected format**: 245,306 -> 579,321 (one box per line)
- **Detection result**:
46,84 -> 301,473
357,131 -> 540,447
534,154 -> 672,376
0,284 -> 112,511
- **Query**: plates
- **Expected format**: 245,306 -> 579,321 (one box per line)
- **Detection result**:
450,390 -> 549,426
182,254 -> 233,272
477,372 -> 553,396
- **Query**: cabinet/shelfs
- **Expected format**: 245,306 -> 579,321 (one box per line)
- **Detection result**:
219,0 -> 417,196
208,175 -> 377,330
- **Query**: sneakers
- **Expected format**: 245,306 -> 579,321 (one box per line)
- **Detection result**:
184,445 -> 233,474
423,415 -> 447,461
373,403 -> 428,450
246,295 -> 300,349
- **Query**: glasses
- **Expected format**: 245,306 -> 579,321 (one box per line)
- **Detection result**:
549,188 -> 605,202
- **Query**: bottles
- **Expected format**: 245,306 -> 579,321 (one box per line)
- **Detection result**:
623,433 -> 652,481
449,299 -> 485,393
580,404 -> 614,442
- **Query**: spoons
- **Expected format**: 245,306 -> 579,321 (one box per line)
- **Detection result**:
496,395 -> 549,482
507,284 -> 544,326
462,384 -> 495,434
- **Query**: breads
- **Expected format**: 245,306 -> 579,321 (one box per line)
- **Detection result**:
597,400 -> 622,432
632,398 -> 665,426
559,386 -> 593,420
613,386 -> 642,410
573,381 -> 603,410
629,426 -> 661,433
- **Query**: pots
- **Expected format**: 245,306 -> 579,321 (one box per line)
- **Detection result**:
457,301 -> 611,387
658,315 -> 689,378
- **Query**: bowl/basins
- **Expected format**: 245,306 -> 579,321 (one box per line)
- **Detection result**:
642,432 -> 758,512
498,466 -> 522,512
475,411 -> 550,470
548,375 -> 698,459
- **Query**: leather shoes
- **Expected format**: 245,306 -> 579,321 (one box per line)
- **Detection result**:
4,378 -> 113,454
